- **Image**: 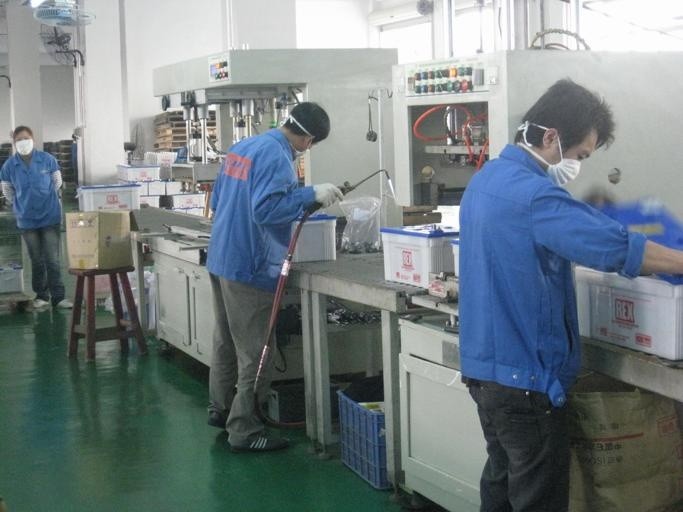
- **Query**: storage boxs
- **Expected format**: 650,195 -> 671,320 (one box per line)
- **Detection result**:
377,222 -> 461,292
568,255 -> 682,370
285,211 -> 337,263
449,237 -> 461,279
70,162 -> 213,228
334,389 -> 394,491
62,210 -> 134,271
0,263 -> 26,296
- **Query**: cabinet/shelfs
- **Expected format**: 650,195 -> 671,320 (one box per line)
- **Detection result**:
147,248 -> 219,369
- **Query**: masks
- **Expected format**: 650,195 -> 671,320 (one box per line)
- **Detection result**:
15,139 -> 34,156
546,136 -> 581,188
290,135 -> 316,157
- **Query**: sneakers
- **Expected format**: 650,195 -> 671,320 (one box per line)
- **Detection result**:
32,298 -> 50,308
230,430 -> 291,453
207,410 -> 226,429
56,298 -> 74,309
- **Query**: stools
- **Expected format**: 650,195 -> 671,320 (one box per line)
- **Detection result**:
64,263 -> 148,367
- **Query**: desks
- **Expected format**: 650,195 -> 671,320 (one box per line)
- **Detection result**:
281,251 -> 381,445
307,254 -> 412,504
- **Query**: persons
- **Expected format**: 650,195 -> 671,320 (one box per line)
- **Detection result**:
205,102 -> 344,453
458,77 -> 683,512
0,126 -> 75,311
583,183 -> 615,219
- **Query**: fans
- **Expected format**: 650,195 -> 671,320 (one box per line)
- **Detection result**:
36,23 -> 84,71
31,0 -> 94,68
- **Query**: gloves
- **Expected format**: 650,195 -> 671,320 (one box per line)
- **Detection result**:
314,182 -> 346,209
